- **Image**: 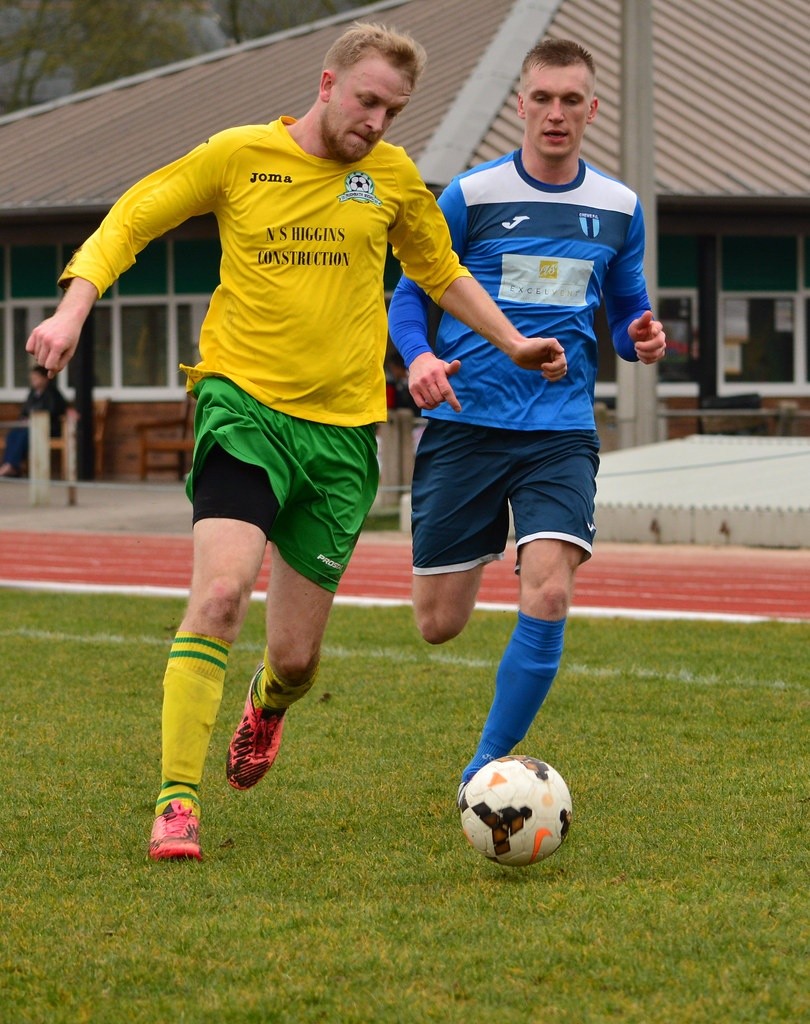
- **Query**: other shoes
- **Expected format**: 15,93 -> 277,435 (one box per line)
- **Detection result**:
0,463 -> 21,477
456,780 -> 468,807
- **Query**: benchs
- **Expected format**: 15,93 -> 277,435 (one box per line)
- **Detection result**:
0,399 -> 111,480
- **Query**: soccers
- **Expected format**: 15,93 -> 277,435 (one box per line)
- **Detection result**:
459,755 -> 573,867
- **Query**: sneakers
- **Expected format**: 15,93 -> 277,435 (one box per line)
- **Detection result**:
226,666 -> 286,789
150,801 -> 204,860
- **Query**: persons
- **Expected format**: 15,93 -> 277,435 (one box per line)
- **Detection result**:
24,22 -> 568,861
387,38 -> 666,806
0,366 -> 65,477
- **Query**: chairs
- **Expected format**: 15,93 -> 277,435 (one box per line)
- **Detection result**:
133,390 -> 198,481
